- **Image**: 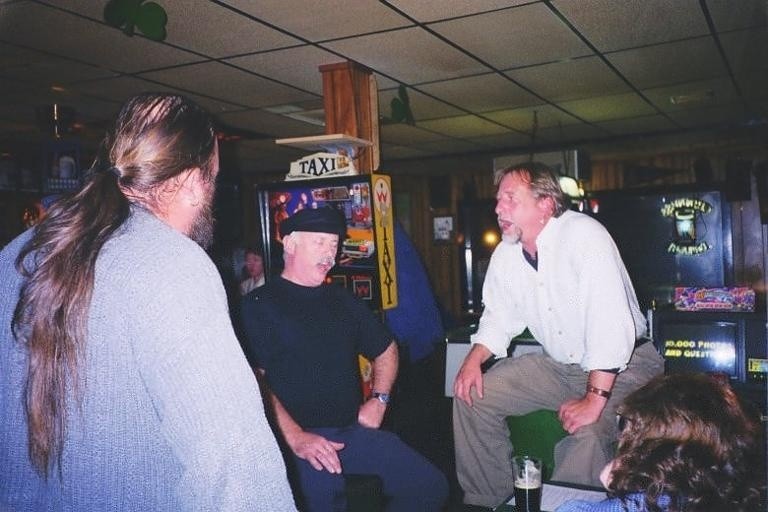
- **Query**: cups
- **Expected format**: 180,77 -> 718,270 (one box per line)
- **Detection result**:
511,455 -> 544,512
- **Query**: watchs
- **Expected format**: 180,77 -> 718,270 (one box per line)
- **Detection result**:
585,384 -> 611,400
371,390 -> 391,404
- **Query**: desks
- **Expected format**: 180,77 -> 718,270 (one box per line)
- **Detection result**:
493,479 -> 610,511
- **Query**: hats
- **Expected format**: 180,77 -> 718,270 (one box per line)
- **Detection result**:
279,208 -> 344,236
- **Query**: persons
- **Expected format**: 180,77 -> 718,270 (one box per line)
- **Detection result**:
0,90 -> 297,511
453,163 -> 665,512
238,241 -> 267,296
232,209 -> 453,511
552,373 -> 768,512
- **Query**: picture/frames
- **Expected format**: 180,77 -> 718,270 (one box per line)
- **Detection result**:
431,215 -> 456,247
